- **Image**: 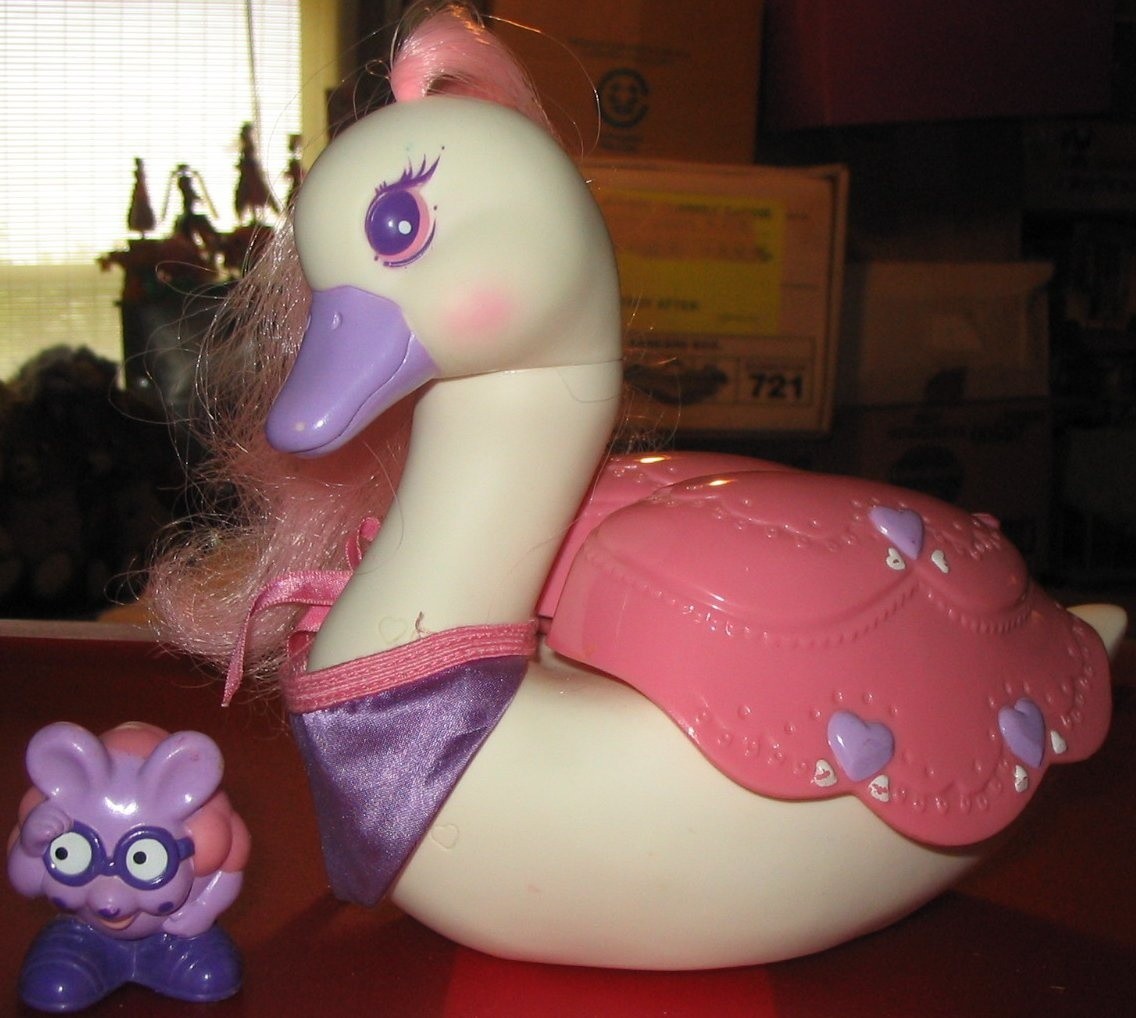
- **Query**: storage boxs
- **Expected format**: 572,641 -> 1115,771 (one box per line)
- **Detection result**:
840,256 -> 1056,405
759,1 -> 1132,117
473,0 -> 764,163
